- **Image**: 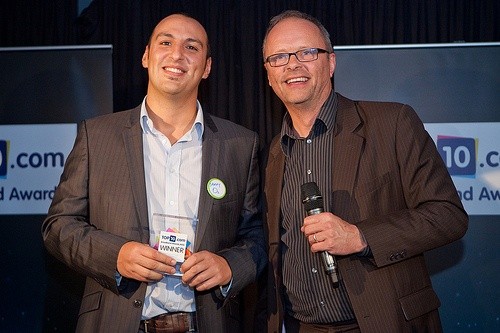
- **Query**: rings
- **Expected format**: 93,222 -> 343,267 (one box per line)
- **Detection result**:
313,233 -> 317,242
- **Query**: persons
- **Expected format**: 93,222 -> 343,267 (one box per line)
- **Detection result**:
41,13 -> 266,333
263,10 -> 468,333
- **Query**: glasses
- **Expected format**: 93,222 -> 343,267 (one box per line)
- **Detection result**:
263,47 -> 331,68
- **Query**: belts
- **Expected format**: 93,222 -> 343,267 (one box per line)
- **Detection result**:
142,314 -> 199,330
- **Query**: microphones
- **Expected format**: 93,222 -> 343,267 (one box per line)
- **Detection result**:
300,182 -> 339,289
